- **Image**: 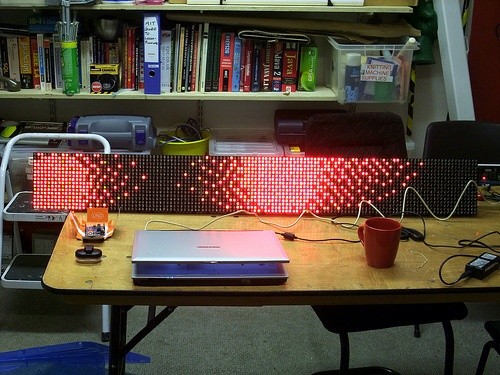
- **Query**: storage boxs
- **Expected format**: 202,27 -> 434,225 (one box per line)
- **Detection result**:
298,40 -> 319,92
325,33 -> 421,105
89,63 -> 121,92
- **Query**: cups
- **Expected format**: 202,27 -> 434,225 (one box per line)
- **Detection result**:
358,218 -> 402,269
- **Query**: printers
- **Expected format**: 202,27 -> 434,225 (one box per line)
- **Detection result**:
67,113 -> 153,151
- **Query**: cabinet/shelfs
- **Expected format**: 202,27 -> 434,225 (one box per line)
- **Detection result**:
0,0 -> 415,153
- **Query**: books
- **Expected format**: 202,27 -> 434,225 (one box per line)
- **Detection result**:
0,16 -> 171,95
171,24 -> 315,93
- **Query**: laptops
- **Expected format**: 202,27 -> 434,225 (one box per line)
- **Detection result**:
132,228 -> 291,284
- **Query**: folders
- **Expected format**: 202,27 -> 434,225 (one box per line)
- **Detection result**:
143,11 -> 160,94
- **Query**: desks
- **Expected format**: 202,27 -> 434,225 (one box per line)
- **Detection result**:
38,186 -> 500,375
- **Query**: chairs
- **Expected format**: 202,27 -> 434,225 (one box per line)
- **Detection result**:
305,113 -> 470,375
414,118 -> 500,339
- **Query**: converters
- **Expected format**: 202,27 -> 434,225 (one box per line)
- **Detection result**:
465,253 -> 499,280
